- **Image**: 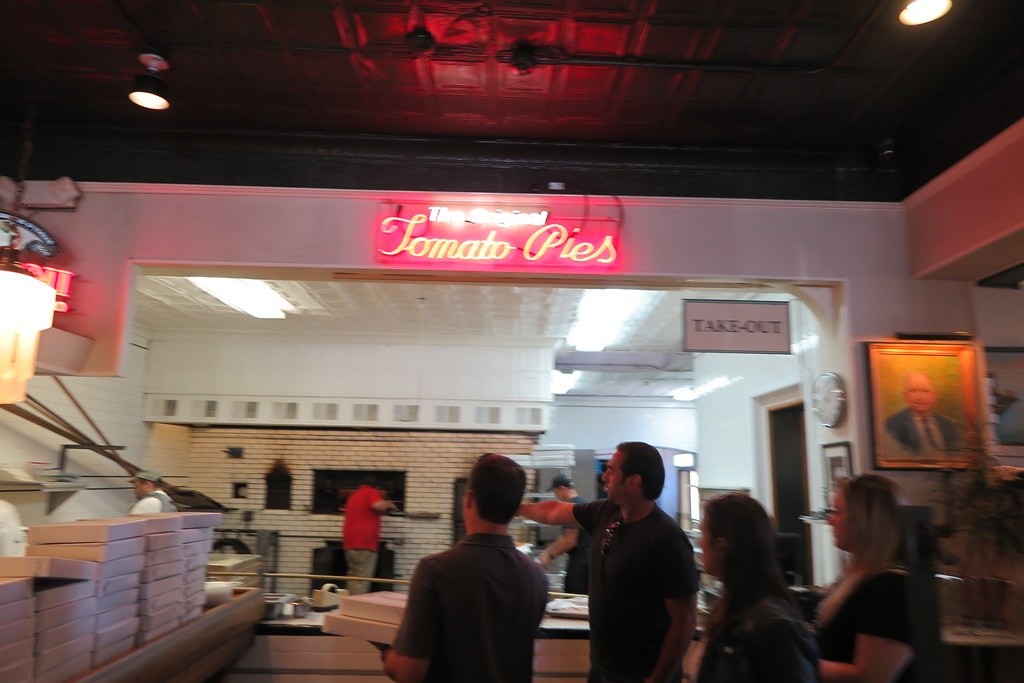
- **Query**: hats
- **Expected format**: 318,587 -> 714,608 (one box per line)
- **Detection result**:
544,474 -> 572,492
129,471 -> 160,485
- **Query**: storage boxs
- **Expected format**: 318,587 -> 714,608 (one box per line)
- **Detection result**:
139,588 -> 183,617
181,606 -> 203,625
137,619 -> 178,647
181,591 -> 207,614
139,603 -> 180,632
155,511 -> 222,530
144,530 -> 185,551
183,566 -> 206,585
182,528 -> 213,544
324,591 -> 408,647
185,539 -> 212,559
0,518 -> 149,683
139,574 -> 184,600
141,560 -> 185,583
184,553 -> 207,573
183,579 -> 205,599
143,544 -> 185,567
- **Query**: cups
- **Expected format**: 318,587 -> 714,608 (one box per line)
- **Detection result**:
294,604 -> 306,617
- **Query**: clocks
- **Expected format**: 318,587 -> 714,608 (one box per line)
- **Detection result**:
811,372 -> 847,428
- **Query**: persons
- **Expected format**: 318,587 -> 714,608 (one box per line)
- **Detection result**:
540,474 -> 590,594
342,480 -> 397,595
128,470 -> 179,515
517,442 -> 696,682
696,493 -> 823,683
817,475 -> 932,682
391,452 -> 549,682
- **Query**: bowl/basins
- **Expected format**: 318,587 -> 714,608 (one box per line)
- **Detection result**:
205,580 -> 242,605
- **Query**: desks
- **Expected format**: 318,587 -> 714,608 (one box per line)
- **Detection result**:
939,622 -> 1024,683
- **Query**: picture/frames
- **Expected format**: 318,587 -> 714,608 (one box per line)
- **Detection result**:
821,441 -> 853,509
864,339 -> 985,473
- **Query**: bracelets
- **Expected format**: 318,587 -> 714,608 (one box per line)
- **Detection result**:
550,553 -> 555,559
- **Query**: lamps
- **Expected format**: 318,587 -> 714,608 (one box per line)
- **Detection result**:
127,47 -> 175,111
